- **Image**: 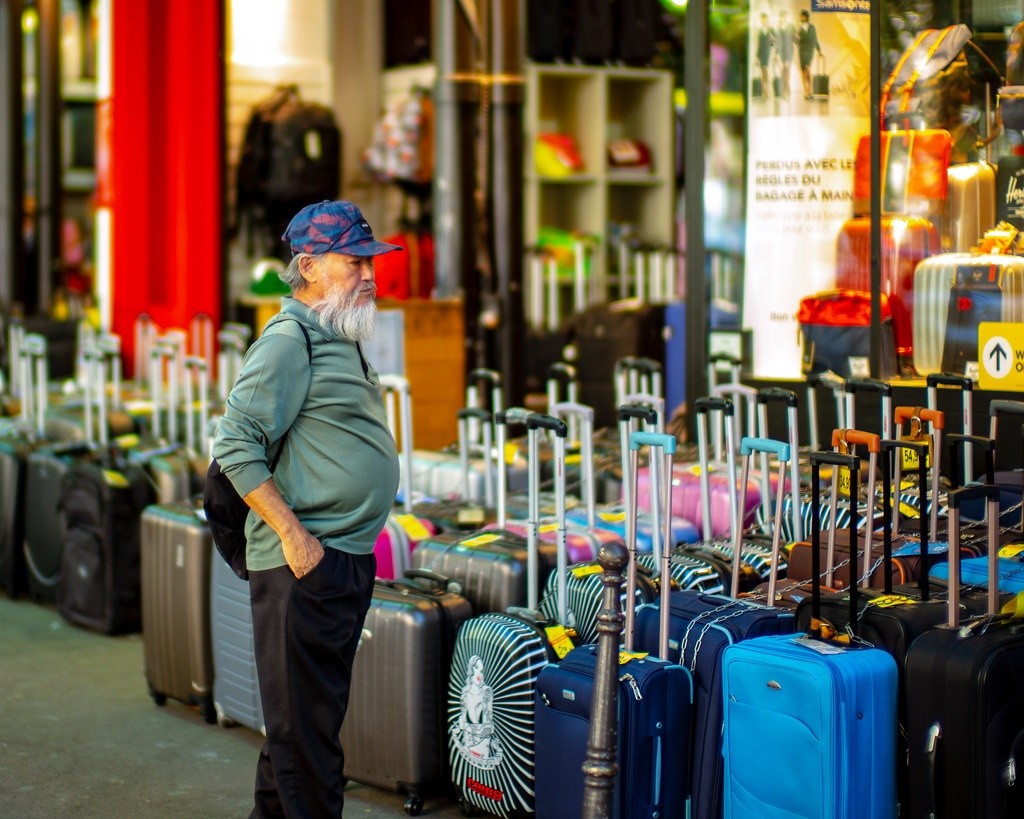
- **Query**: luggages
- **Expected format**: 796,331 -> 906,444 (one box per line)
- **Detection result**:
0,78 -> 1024,819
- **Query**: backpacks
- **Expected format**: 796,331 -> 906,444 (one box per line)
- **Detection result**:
879,23 -> 1002,166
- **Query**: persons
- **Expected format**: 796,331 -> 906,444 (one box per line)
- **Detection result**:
213,198 -> 408,819
757,10 -> 825,101
